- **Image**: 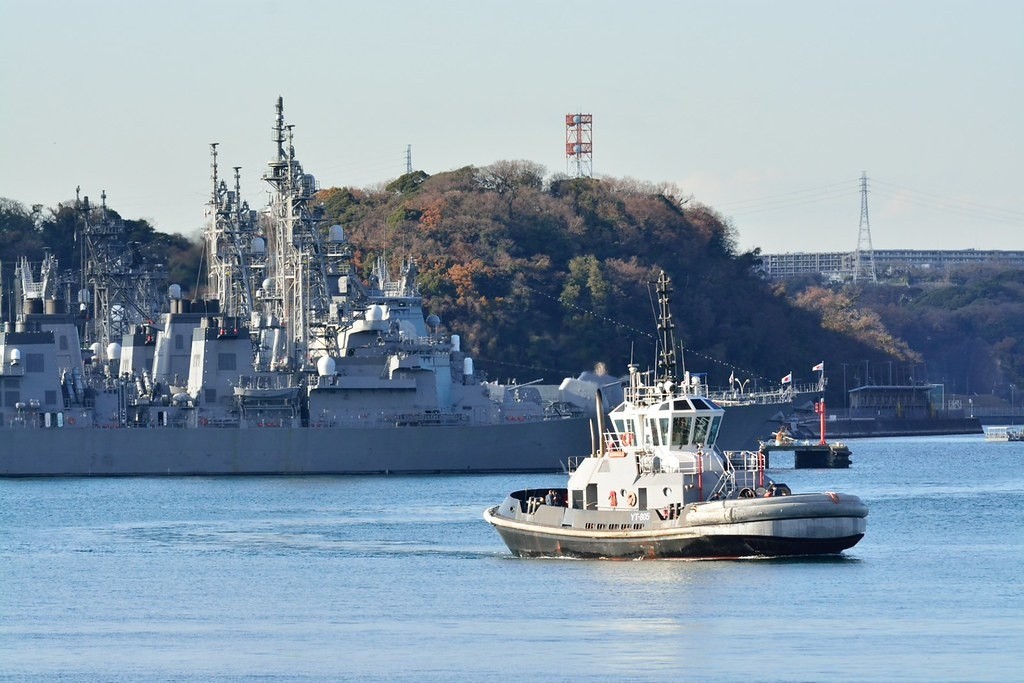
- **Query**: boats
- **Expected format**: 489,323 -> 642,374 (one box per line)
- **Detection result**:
484,269 -> 870,561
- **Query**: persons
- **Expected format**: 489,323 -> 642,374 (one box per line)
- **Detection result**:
545,490 -> 565,507
772,485 -> 782,496
763,489 -> 773,498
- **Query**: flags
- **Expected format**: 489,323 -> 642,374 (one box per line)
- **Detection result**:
812,363 -> 823,371
729,372 -> 733,383
781,374 -> 791,384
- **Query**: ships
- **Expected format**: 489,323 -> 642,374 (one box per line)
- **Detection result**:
0,93 -> 828,478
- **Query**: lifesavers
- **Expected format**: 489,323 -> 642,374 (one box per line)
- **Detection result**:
68,418 -> 76,425
622,432 -> 632,445
627,492 -> 636,506
200,418 -> 208,425
